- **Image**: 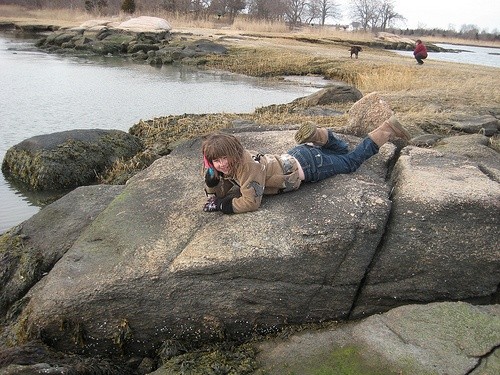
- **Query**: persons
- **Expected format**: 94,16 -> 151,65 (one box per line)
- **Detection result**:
413,39 -> 428,65
201,115 -> 412,214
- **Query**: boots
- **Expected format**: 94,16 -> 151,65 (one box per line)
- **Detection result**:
367,115 -> 410,148
294,121 -> 328,146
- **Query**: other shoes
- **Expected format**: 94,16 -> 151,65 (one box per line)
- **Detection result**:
417,61 -> 424,65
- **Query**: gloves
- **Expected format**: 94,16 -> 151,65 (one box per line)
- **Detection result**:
203,196 -> 224,212
205,169 -> 220,187
